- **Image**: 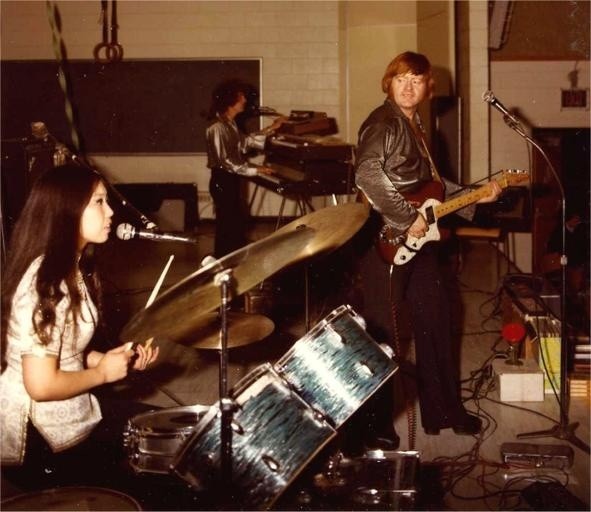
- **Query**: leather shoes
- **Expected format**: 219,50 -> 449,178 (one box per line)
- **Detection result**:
421,410 -> 483,435
370,414 -> 400,450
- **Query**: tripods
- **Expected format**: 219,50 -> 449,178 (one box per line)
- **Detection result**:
517,198 -> 591,455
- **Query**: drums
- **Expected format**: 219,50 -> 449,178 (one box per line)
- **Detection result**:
0,488 -> 141,512
273,306 -> 399,433
170,361 -> 337,510
124,404 -> 211,477
350,451 -> 420,492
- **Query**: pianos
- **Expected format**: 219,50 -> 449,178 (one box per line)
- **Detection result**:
249,133 -> 356,198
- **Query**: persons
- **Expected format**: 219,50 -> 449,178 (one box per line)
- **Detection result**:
205,82 -> 290,259
354,50 -> 503,435
0,166 -> 160,466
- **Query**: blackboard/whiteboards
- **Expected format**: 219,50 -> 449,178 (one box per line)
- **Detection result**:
0,57 -> 263,156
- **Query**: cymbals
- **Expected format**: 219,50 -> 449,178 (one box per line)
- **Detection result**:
167,312 -> 274,351
119,229 -> 316,344
282,202 -> 371,257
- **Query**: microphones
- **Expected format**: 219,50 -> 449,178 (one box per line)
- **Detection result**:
482,90 -> 520,125
116,223 -> 198,246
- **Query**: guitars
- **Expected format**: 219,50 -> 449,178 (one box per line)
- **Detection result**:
377,168 -> 531,266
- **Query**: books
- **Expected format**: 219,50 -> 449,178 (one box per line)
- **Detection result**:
568,333 -> 591,398
